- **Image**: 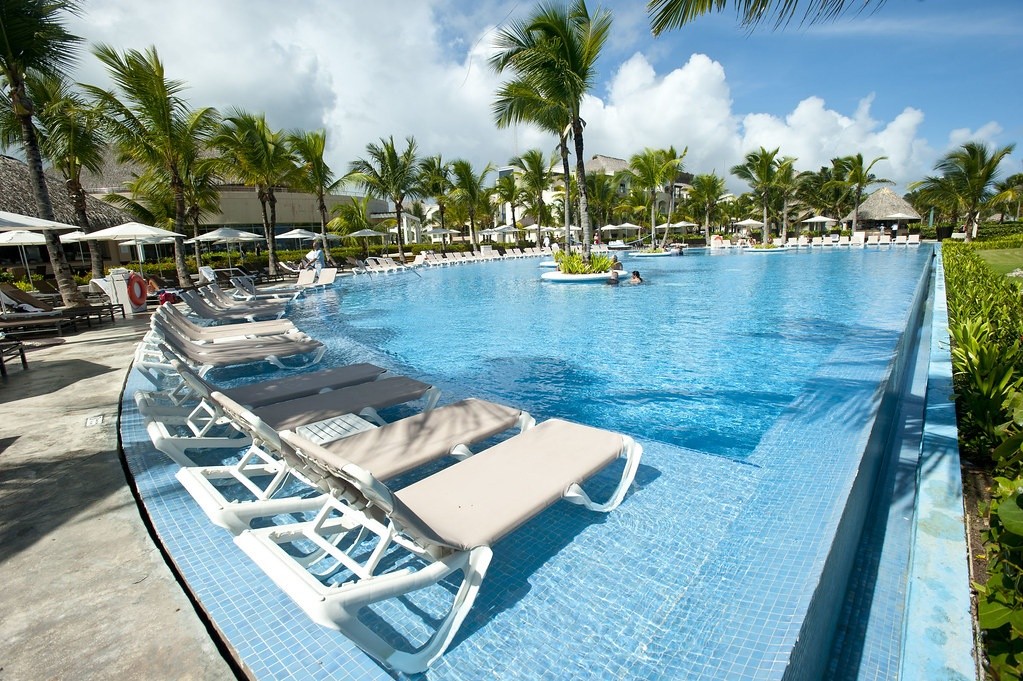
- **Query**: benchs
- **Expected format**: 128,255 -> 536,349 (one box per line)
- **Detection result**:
951,232 -> 967,238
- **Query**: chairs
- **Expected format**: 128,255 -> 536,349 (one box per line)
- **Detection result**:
0,232 -> 920,674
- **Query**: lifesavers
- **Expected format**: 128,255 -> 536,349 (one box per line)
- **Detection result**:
127,274 -> 147,305
714,236 -> 723,245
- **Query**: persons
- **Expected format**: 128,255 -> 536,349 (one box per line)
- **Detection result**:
606,272 -> 620,284
631,271 -> 642,283
299,242 -> 325,278
891,222 -> 898,241
671,247 -> 678,256
679,248 -> 684,256
880,223 -> 885,235
610,255 -> 623,270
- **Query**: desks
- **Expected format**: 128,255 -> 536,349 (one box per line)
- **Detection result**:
212,335 -> 249,342
294,412 -> 376,449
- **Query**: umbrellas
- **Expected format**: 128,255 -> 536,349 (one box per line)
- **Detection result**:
421,226 -> 461,252
654,223 -> 681,240
671,221 -> 699,244
275,229 -> 326,250
801,216 -> 838,237
477,225 -> 521,243
596,223 -> 641,242
0,211 -> 82,231
346,228 -> 389,257
733,218 -> 764,235
0,222 -> 267,287
522,224 -> 582,242
